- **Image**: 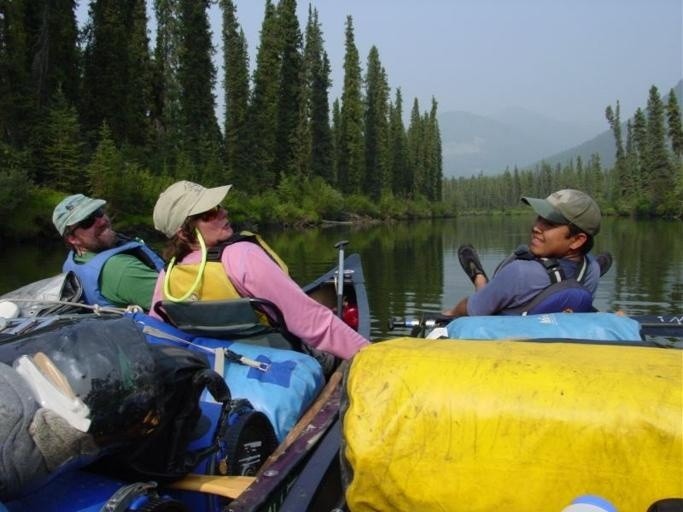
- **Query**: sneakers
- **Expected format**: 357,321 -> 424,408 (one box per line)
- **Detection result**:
457,240 -> 490,284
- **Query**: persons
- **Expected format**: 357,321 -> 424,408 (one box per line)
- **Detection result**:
437,187 -> 613,323
146,178 -> 375,375
49,193 -> 167,314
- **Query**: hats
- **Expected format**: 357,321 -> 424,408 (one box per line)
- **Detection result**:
51,192 -> 108,237
520,187 -> 602,237
152,179 -> 234,238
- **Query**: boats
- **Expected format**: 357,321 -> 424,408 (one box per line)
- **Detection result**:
0,253 -> 371,512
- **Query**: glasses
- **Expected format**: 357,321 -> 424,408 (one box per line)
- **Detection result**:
69,207 -> 105,231
190,203 -> 226,223
